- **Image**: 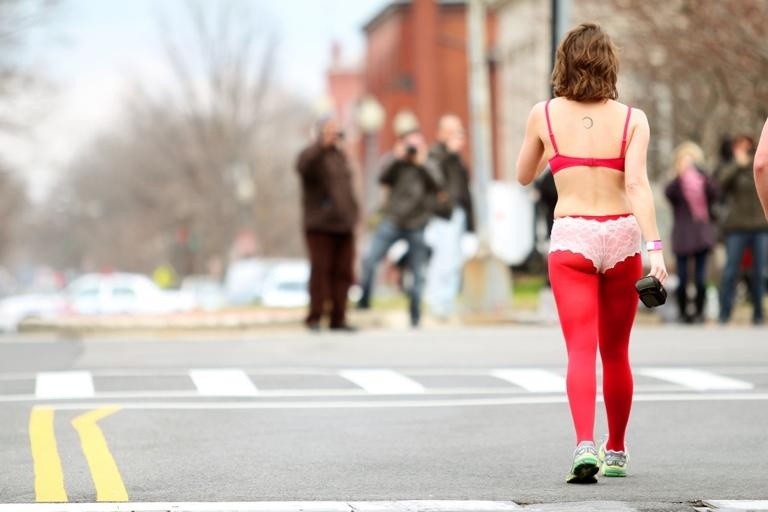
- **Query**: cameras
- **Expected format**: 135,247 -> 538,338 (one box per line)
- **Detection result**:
407,147 -> 417,156
335,132 -> 345,138
746,148 -> 757,156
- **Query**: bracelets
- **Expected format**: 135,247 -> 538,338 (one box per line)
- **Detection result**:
644,239 -> 663,251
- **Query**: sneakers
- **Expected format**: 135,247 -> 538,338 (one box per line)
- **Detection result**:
601,444 -> 630,479
565,440 -> 601,485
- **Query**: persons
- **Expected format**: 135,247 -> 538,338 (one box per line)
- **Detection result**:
751,118 -> 767,220
418,111 -> 478,317
664,139 -> 708,324
513,20 -> 672,486
294,115 -> 359,332
355,108 -> 446,327
708,132 -> 767,325
